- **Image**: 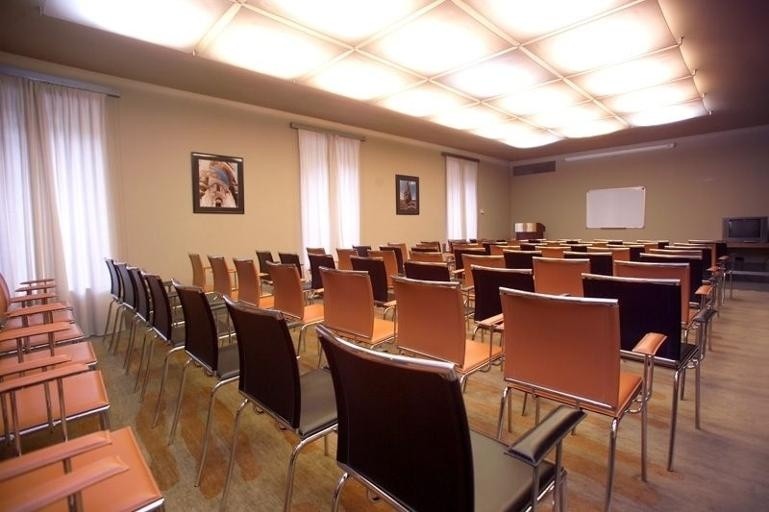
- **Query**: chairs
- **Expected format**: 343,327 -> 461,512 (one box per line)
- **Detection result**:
102,238 -> 730,512
0,276 -> 164,512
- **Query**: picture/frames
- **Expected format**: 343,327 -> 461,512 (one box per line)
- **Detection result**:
191,151 -> 245,214
394,173 -> 420,216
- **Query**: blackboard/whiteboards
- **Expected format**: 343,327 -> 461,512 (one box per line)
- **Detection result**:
586,186 -> 646,230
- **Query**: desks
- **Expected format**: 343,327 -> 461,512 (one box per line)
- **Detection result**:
726,240 -> 769,279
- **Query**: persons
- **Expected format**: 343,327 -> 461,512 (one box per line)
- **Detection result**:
197,160 -> 238,208
399,181 -> 417,211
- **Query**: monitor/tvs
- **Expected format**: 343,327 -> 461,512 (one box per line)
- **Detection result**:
726,217 -> 763,240
